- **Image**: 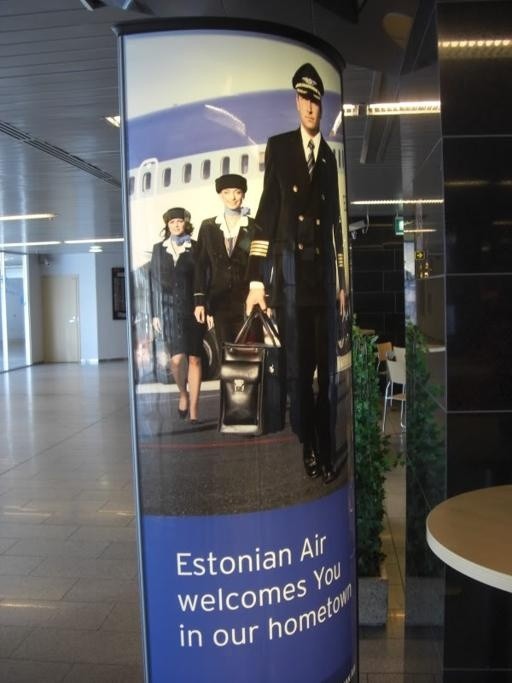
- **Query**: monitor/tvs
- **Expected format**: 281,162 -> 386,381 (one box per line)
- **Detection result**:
394,216 -> 404,236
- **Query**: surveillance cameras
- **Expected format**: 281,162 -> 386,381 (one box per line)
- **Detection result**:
44,257 -> 50,266
347,219 -> 366,233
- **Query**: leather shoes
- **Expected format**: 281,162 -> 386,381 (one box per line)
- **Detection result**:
303,439 -> 338,484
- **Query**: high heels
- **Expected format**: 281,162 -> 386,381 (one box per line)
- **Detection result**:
178,391 -> 200,425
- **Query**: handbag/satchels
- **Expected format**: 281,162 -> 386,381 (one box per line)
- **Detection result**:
218,308 -> 287,438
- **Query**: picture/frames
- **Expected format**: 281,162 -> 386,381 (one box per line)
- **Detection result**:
111,265 -> 127,320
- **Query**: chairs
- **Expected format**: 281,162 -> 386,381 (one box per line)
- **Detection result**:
372,341 -> 405,431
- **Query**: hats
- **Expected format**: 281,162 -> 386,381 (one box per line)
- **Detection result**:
292,63 -> 324,101
163,208 -> 190,223
216,174 -> 246,194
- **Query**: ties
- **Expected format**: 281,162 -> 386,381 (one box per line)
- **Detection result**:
307,140 -> 315,176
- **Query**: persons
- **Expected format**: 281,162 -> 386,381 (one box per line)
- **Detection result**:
245,64 -> 345,483
192,174 -> 256,353
146,208 -> 214,425
